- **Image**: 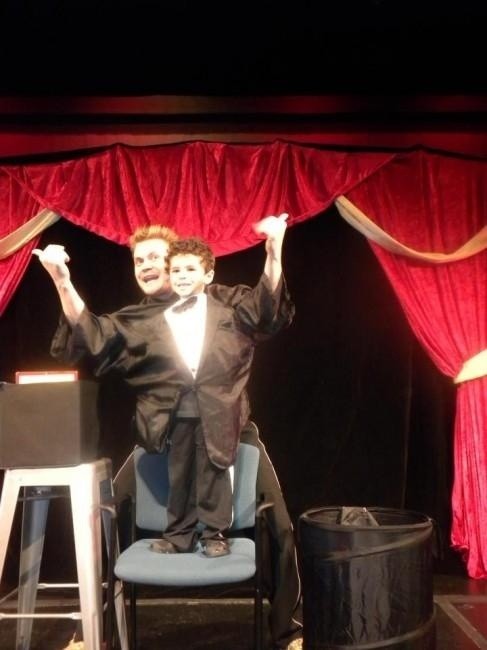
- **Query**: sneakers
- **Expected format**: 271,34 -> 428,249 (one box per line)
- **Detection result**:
64,634 -> 107,650
286,638 -> 304,650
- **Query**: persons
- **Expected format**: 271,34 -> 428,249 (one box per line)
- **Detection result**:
128,241 -> 254,558
31,211 -> 303,650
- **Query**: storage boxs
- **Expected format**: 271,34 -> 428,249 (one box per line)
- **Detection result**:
0,378 -> 100,470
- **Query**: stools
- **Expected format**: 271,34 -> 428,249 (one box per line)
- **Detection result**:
0,457 -> 130,650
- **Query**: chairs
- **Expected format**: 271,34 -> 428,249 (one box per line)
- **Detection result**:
94,417 -> 302,648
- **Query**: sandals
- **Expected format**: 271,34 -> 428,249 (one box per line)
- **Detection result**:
205,539 -> 229,557
151,540 -> 179,553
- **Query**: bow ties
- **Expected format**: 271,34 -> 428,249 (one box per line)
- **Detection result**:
172,297 -> 198,313
